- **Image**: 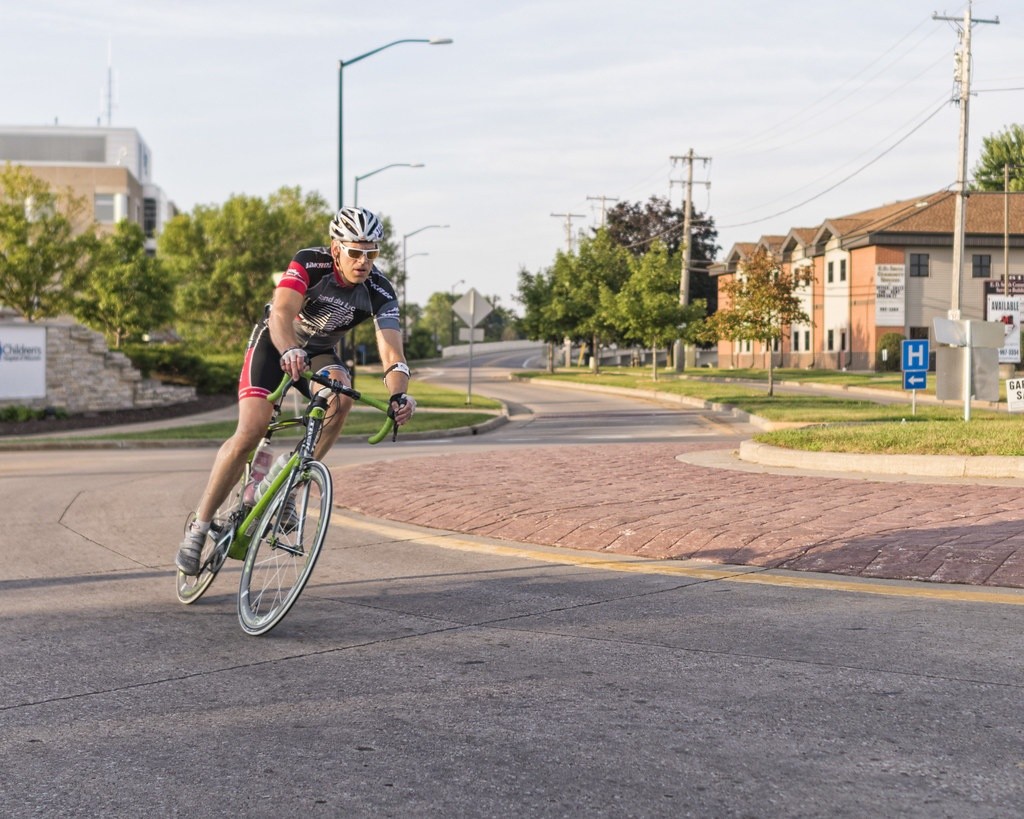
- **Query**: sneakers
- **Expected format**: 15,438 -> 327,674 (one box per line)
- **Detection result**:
275,493 -> 297,526
175,517 -> 207,575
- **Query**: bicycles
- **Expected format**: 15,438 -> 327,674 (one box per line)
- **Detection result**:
174,366 -> 408,636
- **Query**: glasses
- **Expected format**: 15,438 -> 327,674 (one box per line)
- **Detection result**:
334,238 -> 380,260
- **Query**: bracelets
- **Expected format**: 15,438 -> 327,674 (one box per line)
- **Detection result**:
384,362 -> 412,388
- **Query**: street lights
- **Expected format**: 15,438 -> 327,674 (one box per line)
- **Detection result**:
337,37 -> 455,211
355,162 -> 425,208
395,223 -> 452,346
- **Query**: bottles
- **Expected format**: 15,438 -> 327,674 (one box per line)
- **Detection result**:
254,451 -> 293,503
242,441 -> 273,507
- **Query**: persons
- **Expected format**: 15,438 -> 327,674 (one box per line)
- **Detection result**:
175,206 -> 417,576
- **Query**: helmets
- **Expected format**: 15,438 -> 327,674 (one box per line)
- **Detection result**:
329,206 -> 384,243
